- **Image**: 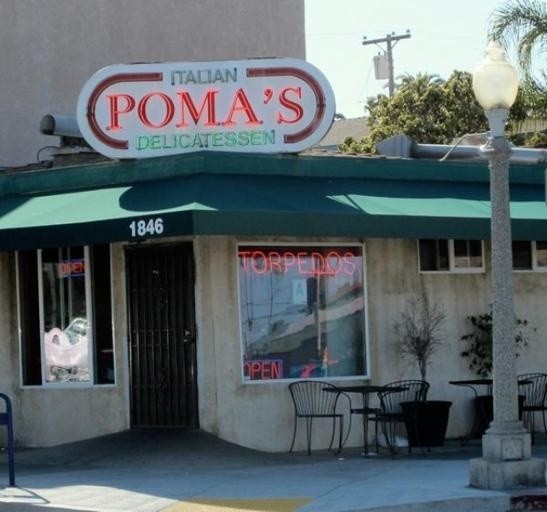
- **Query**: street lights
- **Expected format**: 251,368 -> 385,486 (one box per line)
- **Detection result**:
467,42 -> 546,493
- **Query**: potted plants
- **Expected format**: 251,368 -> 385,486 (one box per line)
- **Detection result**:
461,302 -> 527,422
391,291 -> 452,447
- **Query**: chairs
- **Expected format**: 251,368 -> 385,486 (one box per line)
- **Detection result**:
517,373 -> 547,445
285,380 -> 431,459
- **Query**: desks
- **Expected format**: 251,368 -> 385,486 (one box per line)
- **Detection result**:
449,378 -> 531,448
